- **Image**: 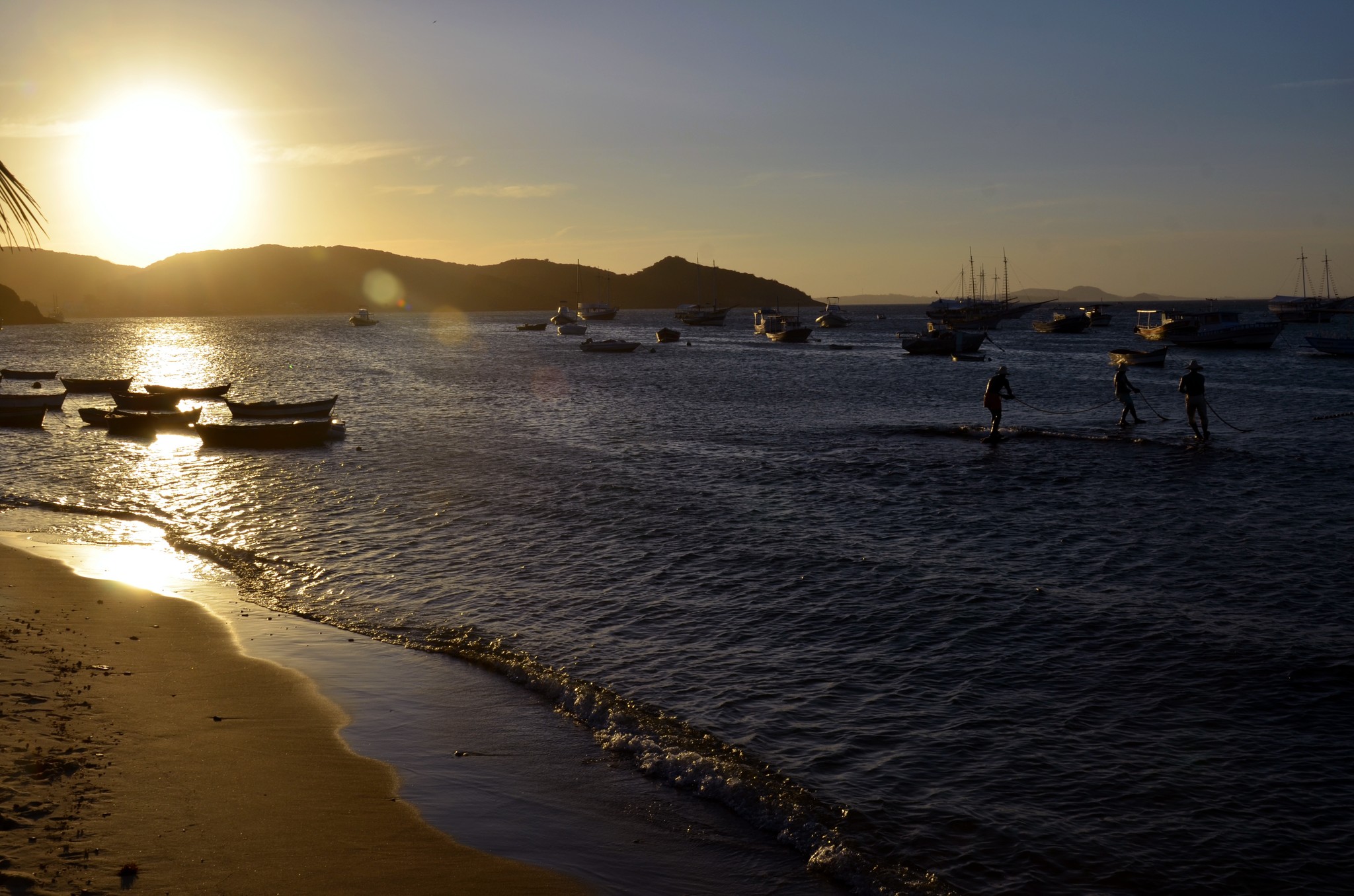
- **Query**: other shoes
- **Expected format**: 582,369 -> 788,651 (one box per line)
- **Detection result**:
1205,431 -> 1210,439
1119,419 -> 1129,425
1194,436 -> 1202,440
989,433 -> 1003,438
1134,419 -> 1146,423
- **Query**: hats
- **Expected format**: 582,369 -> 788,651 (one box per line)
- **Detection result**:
1116,363 -> 1130,371
994,366 -> 1011,376
1184,360 -> 1204,370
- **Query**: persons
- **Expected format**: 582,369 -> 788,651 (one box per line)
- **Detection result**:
1114,362 -> 1145,425
1179,361 -> 1210,439
984,365 -> 1015,437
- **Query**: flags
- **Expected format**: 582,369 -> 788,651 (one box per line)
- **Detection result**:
936,291 -> 938,294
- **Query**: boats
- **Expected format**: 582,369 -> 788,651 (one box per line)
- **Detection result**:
516,322 -> 548,331
815,311 -> 852,328
825,296 -> 840,312
901,321 -> 990,354
876,314 -> 887,319
1,367 -> 59,380
78,382 -> 348,447
951,354 -> 987,362
1108,346 -> 1170,367
550,301 -> 579,325
1031,306 -> 1092,334
655,327 -> 681,342
0,390 -> 70,427
753,296 -> 814,342
59,376 -> 137,393
1304,335 -> 1354,357
1133,298 -> 1288,350
1083,302 -> 1112,326
579,338 -> 641,351
349,305 -> 380,326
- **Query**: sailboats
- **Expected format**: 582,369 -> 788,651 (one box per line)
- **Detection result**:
674,253 -> 741,326
577,265 -> 623,321
925,245 -> 1059,324
558,258 -> 589,335
1267,245 -> 1354,322
